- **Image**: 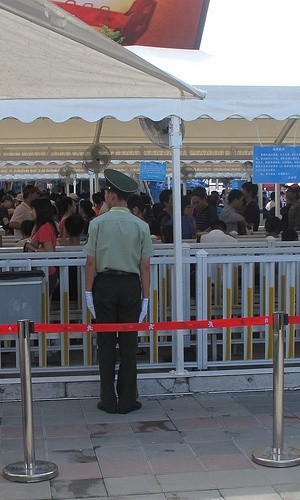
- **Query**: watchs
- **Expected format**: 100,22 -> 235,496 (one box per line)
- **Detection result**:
35,248 -> 38,252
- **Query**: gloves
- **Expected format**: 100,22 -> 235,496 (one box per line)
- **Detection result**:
85,290 -> 96,319
139,297 -> 148,323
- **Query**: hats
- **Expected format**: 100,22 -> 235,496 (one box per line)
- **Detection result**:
103,168 -> 138,193
70,193 -> 79,200
15,194 -> 23,201
3,195 -> 17,201
23,185 -> 46,194
284,184 -> 300,193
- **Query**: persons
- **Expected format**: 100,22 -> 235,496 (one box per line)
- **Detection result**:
83,168 -> 154,413
0,180 -> 300,358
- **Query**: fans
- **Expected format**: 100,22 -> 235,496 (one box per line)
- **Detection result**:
180,166 -> 195,183
83,144 -> 112,173
139,117 -> 185,149
58,165 -> 78,185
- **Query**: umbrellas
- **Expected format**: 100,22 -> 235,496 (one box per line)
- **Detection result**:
0,0 -> 206,100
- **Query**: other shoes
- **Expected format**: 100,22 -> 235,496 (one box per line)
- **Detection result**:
97,401 -> 142,413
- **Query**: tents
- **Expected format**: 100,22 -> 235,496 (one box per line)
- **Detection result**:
0,85 -> 300,376
